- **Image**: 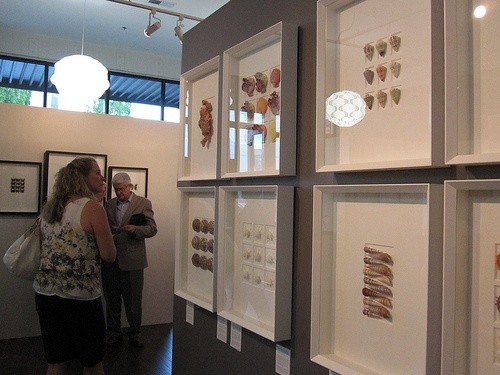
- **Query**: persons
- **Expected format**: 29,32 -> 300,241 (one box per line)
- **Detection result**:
105,173 -> 158,352
33,157 -> 116,375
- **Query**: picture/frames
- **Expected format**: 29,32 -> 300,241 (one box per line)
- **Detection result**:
109,166 -> 148,199
42,150 -> 107,208
0,160 -> 42,214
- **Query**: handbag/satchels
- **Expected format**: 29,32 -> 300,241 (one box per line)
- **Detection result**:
128,213 -> 147,242
3,214 -> 42,281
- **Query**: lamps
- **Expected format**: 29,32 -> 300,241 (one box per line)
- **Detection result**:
175,19 -> 183,42
144,9 -> 161,38
50,0 -> 110,98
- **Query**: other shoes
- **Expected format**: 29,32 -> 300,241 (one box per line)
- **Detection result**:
128,335 -> 145,347
104,335 -> 124,347
